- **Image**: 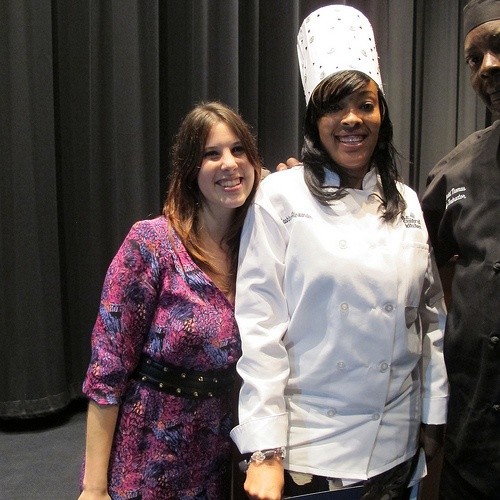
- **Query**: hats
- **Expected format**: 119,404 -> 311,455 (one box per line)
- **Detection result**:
296,5 -> 385,107
463,0 -> 500,40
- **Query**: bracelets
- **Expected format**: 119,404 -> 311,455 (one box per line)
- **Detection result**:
238,447 -> 287,473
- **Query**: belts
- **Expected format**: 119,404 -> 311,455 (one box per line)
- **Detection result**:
132,352 -> 233,400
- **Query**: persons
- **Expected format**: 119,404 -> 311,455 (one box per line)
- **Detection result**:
254,0 -> 500,500
229,4 -> 449,500
78,100 -> 261,500
